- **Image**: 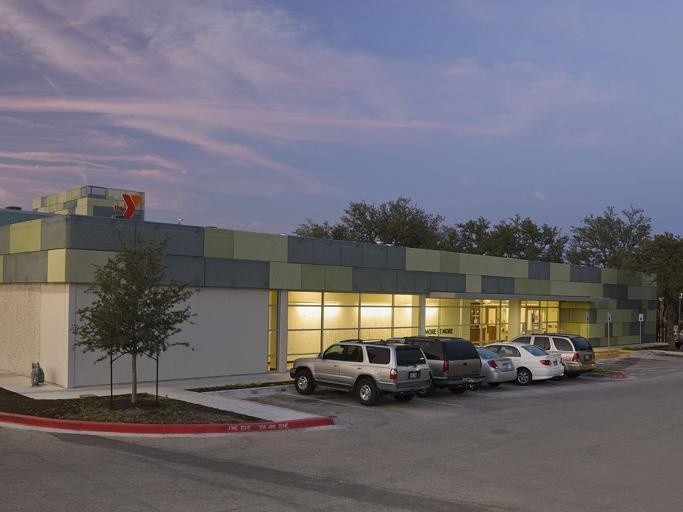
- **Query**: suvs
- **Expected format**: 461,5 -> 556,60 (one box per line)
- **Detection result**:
512,333 -> 596,379
385,334 -> 481,399
288,337 -> 433,406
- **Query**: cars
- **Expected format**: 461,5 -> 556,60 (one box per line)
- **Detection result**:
485,342 -> 565,387
474,345 -> 517,390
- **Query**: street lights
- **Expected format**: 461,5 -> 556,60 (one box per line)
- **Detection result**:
658,296 -> 664,342
678,292 -> 682,331
